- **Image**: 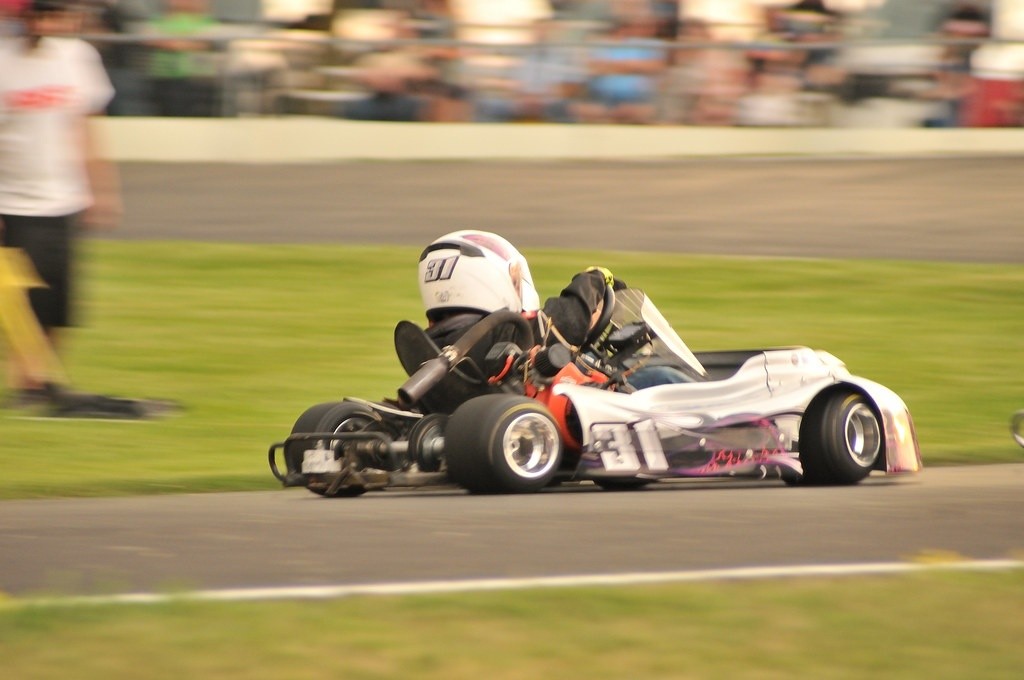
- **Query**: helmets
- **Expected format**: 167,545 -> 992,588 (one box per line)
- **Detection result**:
416,229 -> 540,319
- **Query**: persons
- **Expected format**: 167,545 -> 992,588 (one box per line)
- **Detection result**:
416,227 -> 700,395
1,1 -> 121,403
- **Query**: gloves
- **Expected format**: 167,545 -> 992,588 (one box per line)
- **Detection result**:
586,265 -> 626,291
